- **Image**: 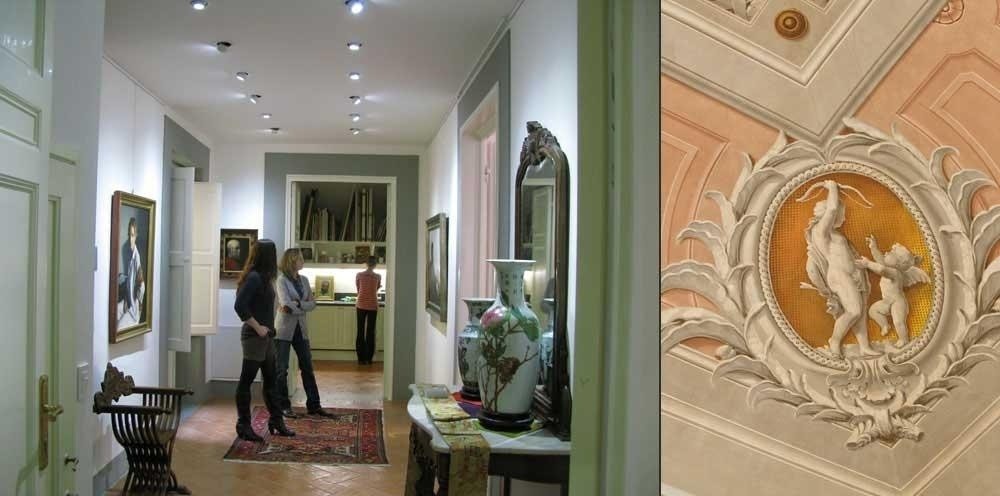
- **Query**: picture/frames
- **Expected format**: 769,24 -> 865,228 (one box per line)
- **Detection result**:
426,214 -> 447,322
109,191 -> 156,344
219,230 -> 258,279
314,275 -> 335,302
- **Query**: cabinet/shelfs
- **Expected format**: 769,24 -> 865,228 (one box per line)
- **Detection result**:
305,304 -> 386,352
295,239 -> 386,269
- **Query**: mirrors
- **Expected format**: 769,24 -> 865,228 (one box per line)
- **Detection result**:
514,120 -> 571,441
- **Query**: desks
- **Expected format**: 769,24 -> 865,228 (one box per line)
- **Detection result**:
406,383 -> 570,496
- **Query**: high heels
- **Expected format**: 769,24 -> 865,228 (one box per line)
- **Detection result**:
267,419 -> 296,436
236,423 -> 263,441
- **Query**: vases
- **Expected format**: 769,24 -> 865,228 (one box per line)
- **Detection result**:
458,298 -> 496,399
476,259 -> 540,432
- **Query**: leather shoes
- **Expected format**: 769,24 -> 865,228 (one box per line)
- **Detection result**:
308,409 -> 334,418
282,408 -> 297,418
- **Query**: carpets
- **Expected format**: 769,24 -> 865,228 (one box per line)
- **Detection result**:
222,406 -> 388,465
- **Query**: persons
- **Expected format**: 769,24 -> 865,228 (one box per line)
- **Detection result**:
274,248 -> 333,418
356,256 -> 381,365
118,218 -> 146,331
804,180 -> 884,360
225,239 -> 242,271
233,239 -> 295,442
855,234 -> 932,348
